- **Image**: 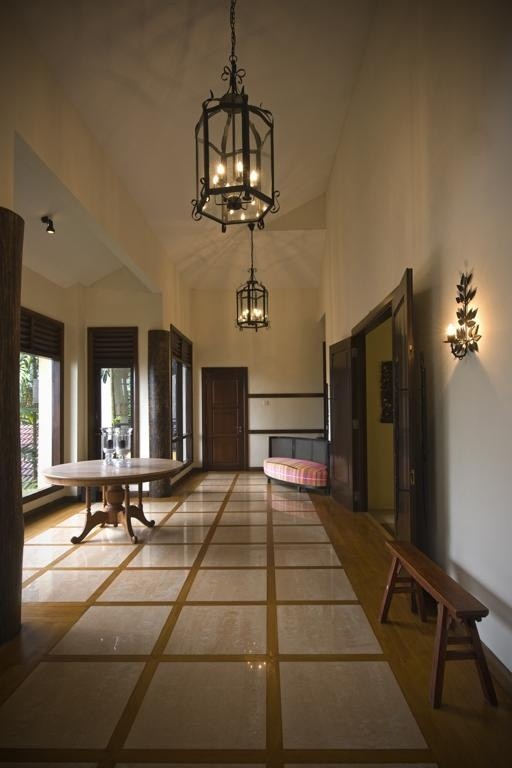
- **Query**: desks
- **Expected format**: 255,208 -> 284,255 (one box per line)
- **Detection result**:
44,457 -> 184,545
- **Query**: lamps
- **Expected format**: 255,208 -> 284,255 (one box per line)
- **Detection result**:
236,222 -> 269,332
41,216 -> 55,235
191,1 -> 281,233
442,272 -> 481,361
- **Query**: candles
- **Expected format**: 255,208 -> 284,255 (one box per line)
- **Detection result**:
107,440 -> 113,449
119,439 -> 126,450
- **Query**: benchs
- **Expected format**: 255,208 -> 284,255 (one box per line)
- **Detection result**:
379,539 -> 499,710
263,457 -> 329,494
